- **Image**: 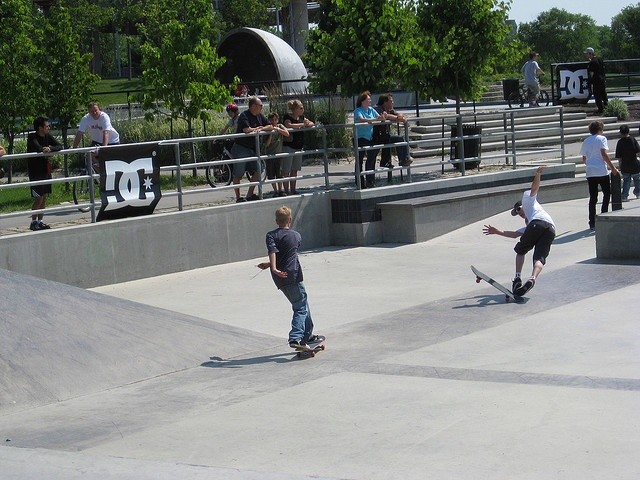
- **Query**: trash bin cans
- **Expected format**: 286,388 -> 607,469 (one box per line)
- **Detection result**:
450,125 -> 483,170
503,78 -> 519,100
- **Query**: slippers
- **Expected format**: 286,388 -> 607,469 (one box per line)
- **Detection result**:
236,197 -> 247,202
246,194 -> 260,201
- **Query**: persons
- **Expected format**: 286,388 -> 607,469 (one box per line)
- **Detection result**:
614,124 -> 640,202
520,51 -> 545,108
72,101 -> 120,167
282,100 -> 315,194
229,97 -> 273,203
482,166 -> 557,299
212,104 -> 241,144
583,46 -> 609,116
0,144 -> 6,180
261,111 -> 290,197
26,116 -> 64,231
350,90 -> 387,189
372,93 -> 414,170
256,206 -> 313,351
579,120 -> 618,230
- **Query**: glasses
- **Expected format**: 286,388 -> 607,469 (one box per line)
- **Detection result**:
256,104 -> 263,107
515,206 -> 522,214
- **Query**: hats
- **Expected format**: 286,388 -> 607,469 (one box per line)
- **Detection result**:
620,125 -> 629,132
529,51 -> 539,57
511,201 -> 522,216
583,47 -> 594,54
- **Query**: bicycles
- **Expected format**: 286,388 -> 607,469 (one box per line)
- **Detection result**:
508,74 -> 549,109
206,138 -> 267,188
73,146 -> 101,213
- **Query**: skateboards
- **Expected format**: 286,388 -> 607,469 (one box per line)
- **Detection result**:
470,265 -> 523,303
295,335 -> 326,357
611,171 -> 622,211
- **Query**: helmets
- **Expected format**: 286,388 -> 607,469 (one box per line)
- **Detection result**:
226,104 -> 238,112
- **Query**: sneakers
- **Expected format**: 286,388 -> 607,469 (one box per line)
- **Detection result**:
515,279 -> 534,296
365,181 -> 377,188
303,335 -> 318,344
38,222 -> 50,229
290,339 -> 310,349
512,277 -> 522,294
30,221 -> 41,231
380,161 -> 394,168
356,181 -> 368,189
